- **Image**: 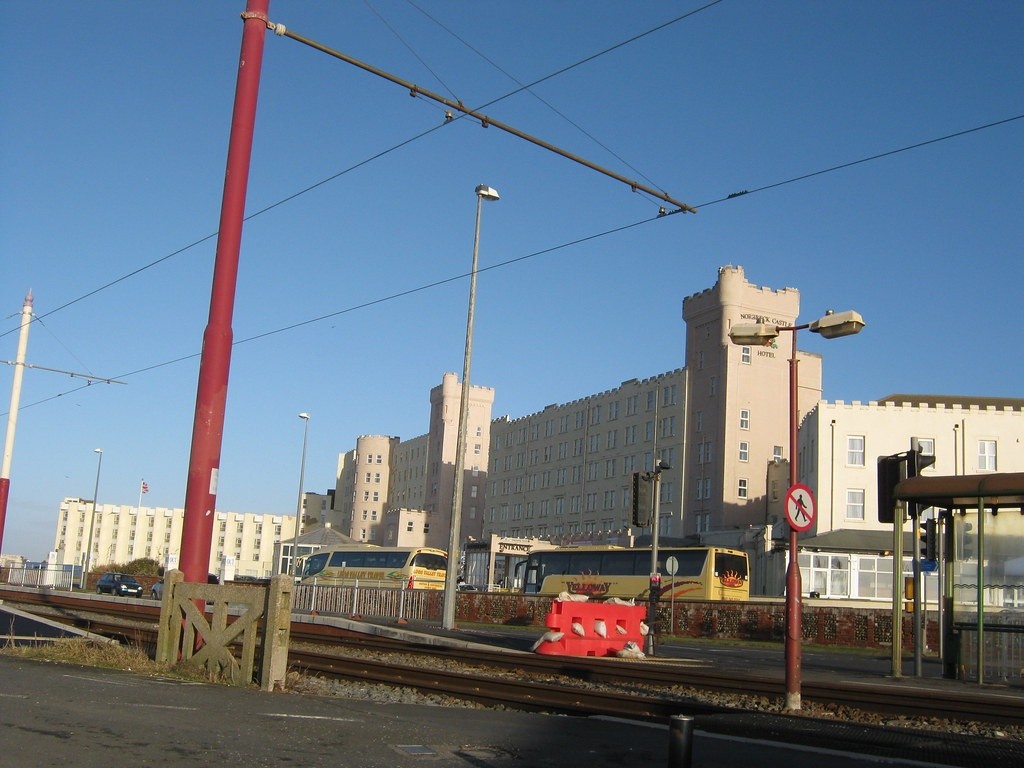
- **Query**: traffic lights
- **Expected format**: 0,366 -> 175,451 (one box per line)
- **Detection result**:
952,513 -> 973,562
908,450 -> 936,517
921,517 -> 936,563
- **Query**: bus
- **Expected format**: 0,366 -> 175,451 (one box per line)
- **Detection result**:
297,543 -> 448,591
513,543 -> 751,605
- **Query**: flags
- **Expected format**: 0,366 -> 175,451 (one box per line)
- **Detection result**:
142,482 -> 149,493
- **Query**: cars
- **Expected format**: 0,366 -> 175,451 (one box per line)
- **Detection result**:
95,572 -> 143,599
151,574 -> 228,606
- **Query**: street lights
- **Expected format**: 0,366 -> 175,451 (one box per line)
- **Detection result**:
438,184 -> 501,630
725,308 -> 867,704
288,411 -> 313,577
80,448 -> 104,590
